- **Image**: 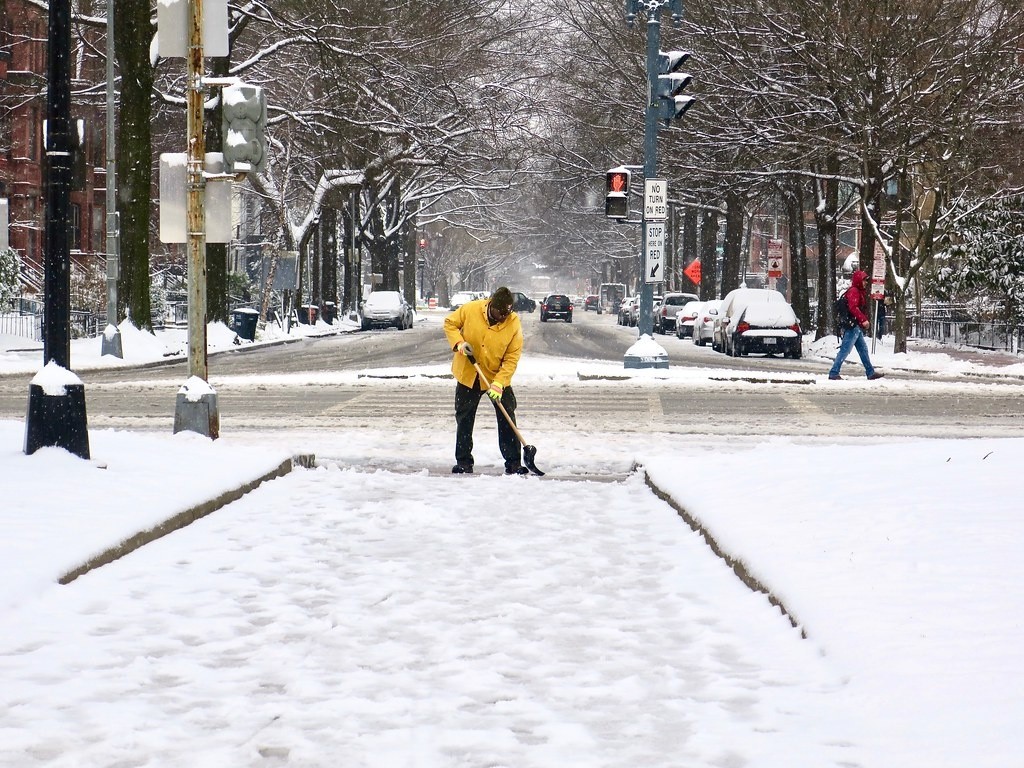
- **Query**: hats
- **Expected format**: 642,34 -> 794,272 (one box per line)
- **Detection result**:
491,287 -> 515,309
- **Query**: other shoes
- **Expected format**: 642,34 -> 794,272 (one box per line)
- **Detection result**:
505,461 -> 529,474
829,375 -> 843,380
868,372 -> 884,380
452,464 -> 473,474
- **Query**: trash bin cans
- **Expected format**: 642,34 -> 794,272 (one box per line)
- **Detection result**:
299,304 -> 319,326
232,308 -> 260,343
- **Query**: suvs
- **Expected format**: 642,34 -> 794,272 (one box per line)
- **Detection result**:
656,293 -> 701,335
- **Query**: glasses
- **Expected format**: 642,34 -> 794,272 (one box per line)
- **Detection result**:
496,306 -> 513,317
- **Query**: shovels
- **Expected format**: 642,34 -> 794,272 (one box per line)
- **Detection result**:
465,346 -> 545,476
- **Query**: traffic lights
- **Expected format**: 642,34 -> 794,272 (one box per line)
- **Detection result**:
606,171 -> 629,194
606,196 -> 630,219
660,50 -> 697,120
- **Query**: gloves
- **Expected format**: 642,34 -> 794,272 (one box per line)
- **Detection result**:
457,340 -> 473,357
486,382 -> 503,402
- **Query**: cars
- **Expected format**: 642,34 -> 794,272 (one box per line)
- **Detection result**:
451,290 -> 491,311
692,300 -> 726,346
584,295 -> 599,312
539,294 -> 574,323
511,292 -> 537,314
574,297 -> 587,309
358,290 -> 414,331
617,294 -> 664,327
709,289 -> 804,359
675,301 -> 708,339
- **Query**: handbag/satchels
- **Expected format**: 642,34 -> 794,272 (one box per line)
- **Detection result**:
834,287 -> 863,330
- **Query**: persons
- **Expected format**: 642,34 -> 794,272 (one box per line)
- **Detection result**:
443,287 -> 529,475
828,270 -> 885,380
